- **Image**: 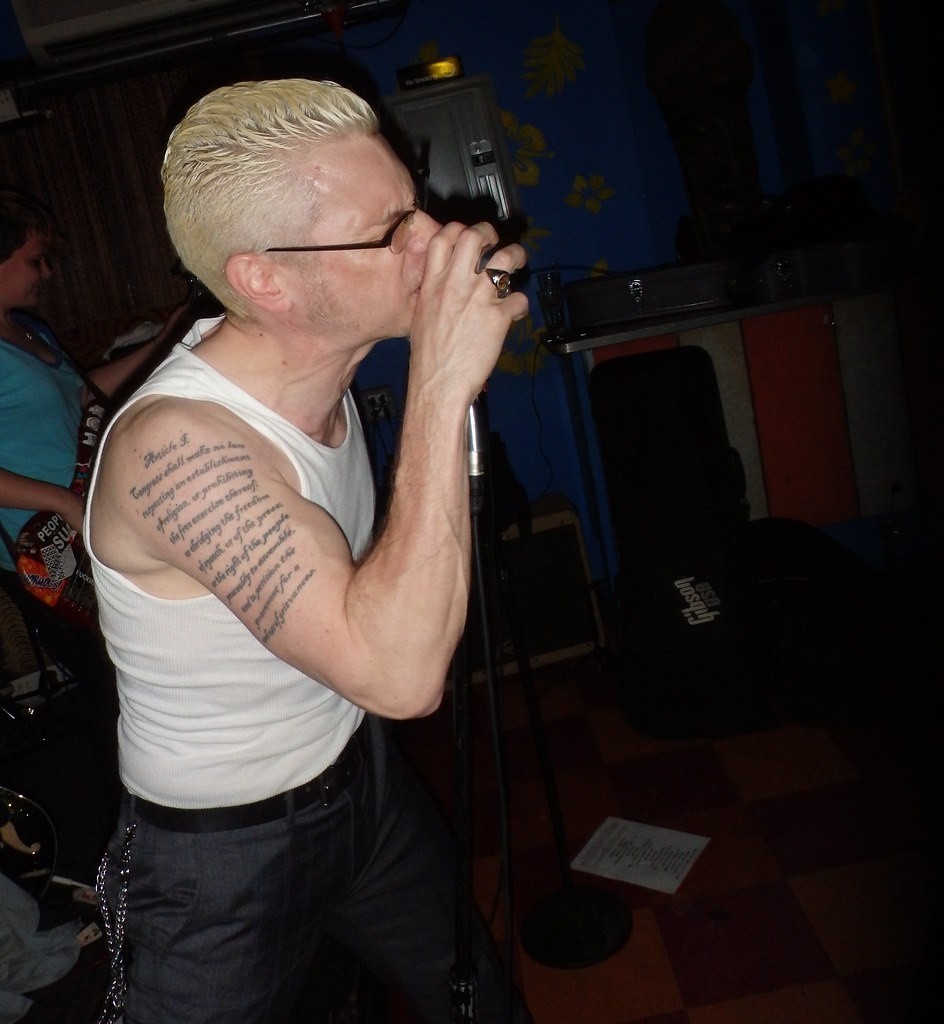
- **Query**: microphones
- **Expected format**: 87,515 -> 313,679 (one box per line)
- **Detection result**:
465,243 -> 512,513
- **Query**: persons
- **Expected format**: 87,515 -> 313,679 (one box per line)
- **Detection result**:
82,78 -> 535,1024
0,205 -> 189,712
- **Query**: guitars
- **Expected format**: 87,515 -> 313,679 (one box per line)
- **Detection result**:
0,727 -> 69,896
14,396 -> 117,640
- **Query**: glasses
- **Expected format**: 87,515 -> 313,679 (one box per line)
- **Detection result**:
255,168 -> 430,255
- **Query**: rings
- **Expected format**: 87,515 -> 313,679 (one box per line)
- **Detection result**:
481,267 -> 513,295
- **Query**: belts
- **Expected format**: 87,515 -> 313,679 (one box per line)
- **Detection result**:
133,712 -> 369,833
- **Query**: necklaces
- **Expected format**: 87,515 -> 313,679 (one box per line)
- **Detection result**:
19,329 -> 32,345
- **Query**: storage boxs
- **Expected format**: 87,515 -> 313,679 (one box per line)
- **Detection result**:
442,493 -> 605,691
576,258 -> 731,326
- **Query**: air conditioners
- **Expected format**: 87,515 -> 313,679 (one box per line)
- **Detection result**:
12,0 -> 337,68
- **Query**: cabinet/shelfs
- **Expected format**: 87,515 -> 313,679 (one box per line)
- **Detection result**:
538,287 -> 918,529
